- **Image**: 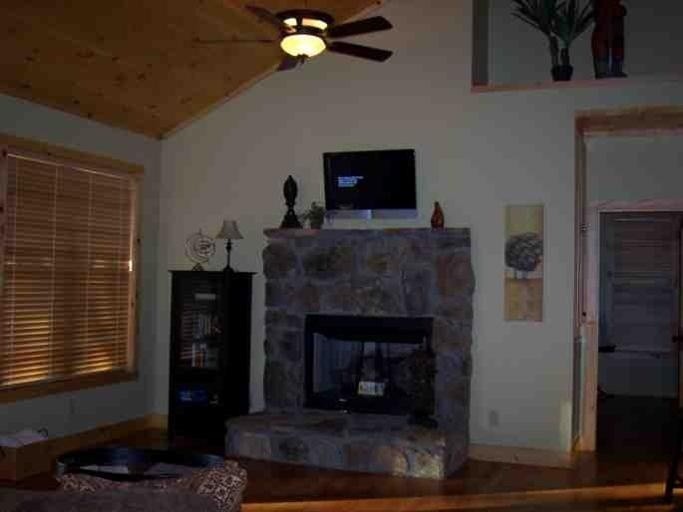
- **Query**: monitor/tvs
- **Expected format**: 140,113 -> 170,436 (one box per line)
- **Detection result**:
322,147 -> 421,222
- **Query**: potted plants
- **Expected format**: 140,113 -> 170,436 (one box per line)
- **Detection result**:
302,201 -> 326,229
511,1 -> 596,82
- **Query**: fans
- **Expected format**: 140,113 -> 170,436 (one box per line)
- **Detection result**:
193,5 -> 393,73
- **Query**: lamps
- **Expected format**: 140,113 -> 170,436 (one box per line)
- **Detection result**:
215,219 -> 243,272
278,9 -> 332,66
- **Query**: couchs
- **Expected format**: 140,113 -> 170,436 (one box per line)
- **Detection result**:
0,460 -> 247,512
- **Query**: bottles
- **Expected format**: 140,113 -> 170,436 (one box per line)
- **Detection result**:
431,200 -> 444,230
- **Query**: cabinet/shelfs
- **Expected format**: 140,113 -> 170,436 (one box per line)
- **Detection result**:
166,269 -> 258,457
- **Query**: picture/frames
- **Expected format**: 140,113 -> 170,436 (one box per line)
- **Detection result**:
469,1 -> 630,94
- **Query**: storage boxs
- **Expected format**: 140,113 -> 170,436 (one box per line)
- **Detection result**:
0,438 -> 51,480
